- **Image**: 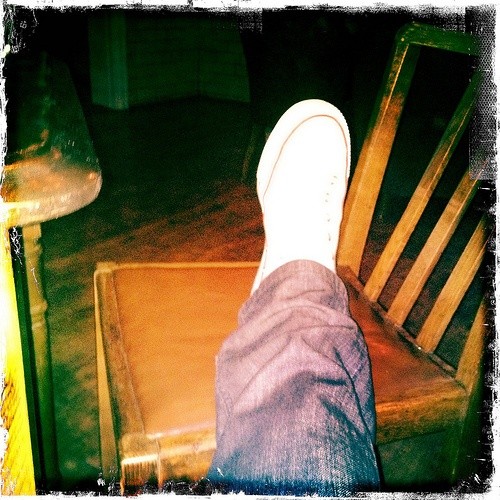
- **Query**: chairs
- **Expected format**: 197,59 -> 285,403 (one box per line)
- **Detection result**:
92,21 -> 494,492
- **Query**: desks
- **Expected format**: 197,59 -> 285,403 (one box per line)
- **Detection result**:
4,132 -> 106,492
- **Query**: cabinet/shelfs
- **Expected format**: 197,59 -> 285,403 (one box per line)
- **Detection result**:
85,10 -> 251,110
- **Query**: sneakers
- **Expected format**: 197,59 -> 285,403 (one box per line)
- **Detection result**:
248,98 -> 352,301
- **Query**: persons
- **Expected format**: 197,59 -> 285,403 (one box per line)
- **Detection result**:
209,97 -> 385,490
